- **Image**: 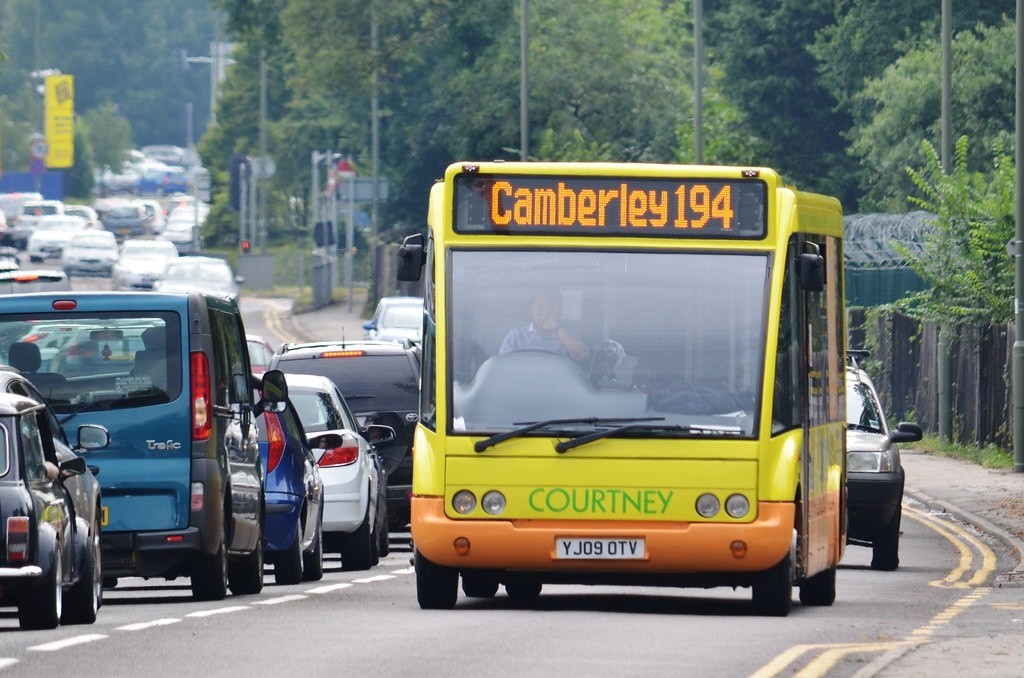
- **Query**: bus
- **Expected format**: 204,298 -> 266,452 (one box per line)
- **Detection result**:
395,160 -> 849,619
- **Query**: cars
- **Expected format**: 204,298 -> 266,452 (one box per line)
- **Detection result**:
242,372 -> 343,587
22,200 -> 64,219
0,322 -> 157,628
0,210 -> 72,291
95,144 -> 212,250
27,215 -> 92,263
62,230 -> 120,278
152,255 -> 245,308
243,334 -> 275,381
110,239 -> 179,292
363,295 -> 425,352
65,205 -> 105,230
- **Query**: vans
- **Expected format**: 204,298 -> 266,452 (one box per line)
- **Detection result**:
0,291 -> 290,601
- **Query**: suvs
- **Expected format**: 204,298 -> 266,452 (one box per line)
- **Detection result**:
842,350 -> 923,571
251,370 -> 398,571
262,339 -> 427,532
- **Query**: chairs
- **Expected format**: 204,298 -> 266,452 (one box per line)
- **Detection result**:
129,325 -> 166,387
6,341 -> 69,393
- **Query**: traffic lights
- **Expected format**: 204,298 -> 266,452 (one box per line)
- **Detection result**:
242,240 -> 252,253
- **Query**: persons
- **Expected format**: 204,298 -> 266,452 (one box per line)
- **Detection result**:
499,286 -> 590,363
45,461 -> 59,479
